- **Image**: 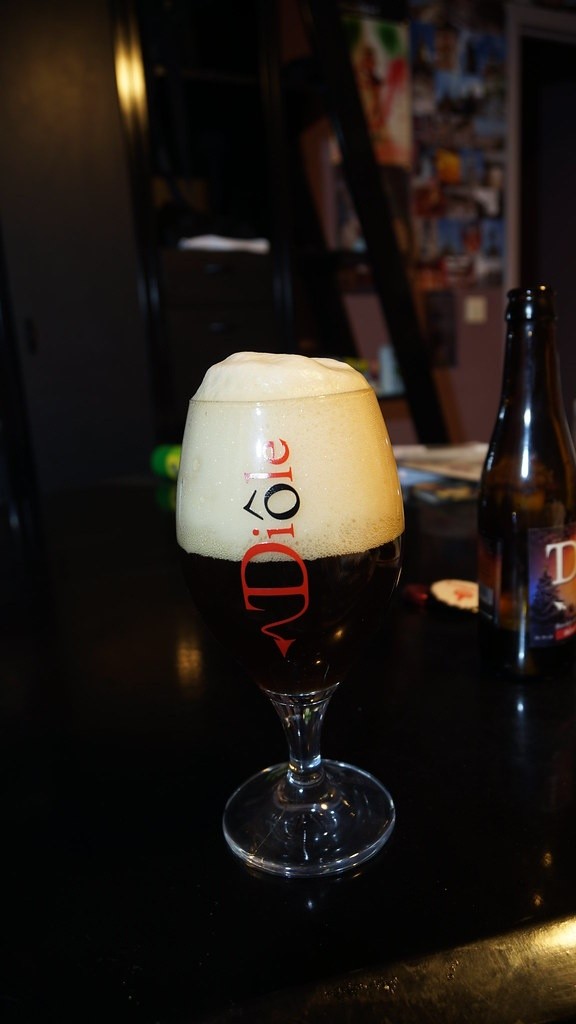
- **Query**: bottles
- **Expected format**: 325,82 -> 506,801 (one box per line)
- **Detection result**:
478,284 -> 576,645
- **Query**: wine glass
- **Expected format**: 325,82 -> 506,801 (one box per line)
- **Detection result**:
177,381 -> 405,875
